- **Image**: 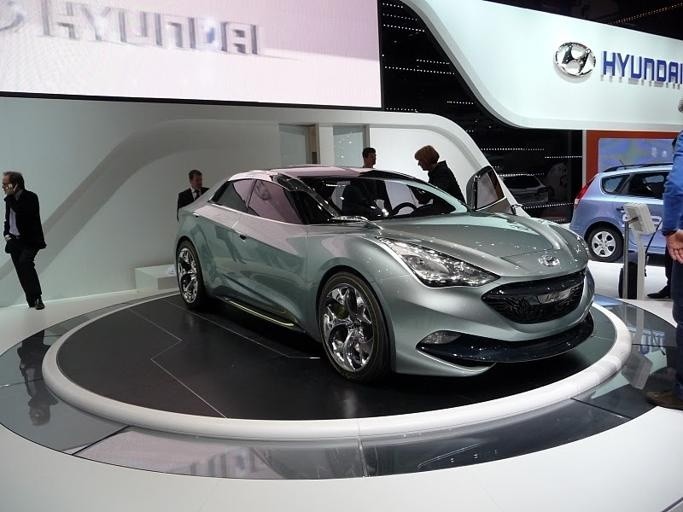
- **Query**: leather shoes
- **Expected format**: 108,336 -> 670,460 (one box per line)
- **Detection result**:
647,282 -> 671,300
35,297 -> 45,310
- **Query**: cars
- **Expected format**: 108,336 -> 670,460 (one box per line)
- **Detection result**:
173,166 -> 595,383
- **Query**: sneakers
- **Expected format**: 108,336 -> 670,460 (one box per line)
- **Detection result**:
645,390 -> 683,410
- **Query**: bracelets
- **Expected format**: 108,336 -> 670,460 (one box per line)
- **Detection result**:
661,230 -> 676,236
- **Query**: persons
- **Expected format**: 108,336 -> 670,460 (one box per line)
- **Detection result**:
646,134 -> 676,299
413,144 -> 466,215
644,129 -> 682,413
362,144 -> 392,215
1,170 -> 48,311
15,330 -> 59,426
174,168 -> 210,221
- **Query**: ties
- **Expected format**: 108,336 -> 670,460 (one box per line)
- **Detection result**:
195,189 -> 200,200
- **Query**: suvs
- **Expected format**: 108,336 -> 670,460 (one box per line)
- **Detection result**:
568,162 -> 673,261
